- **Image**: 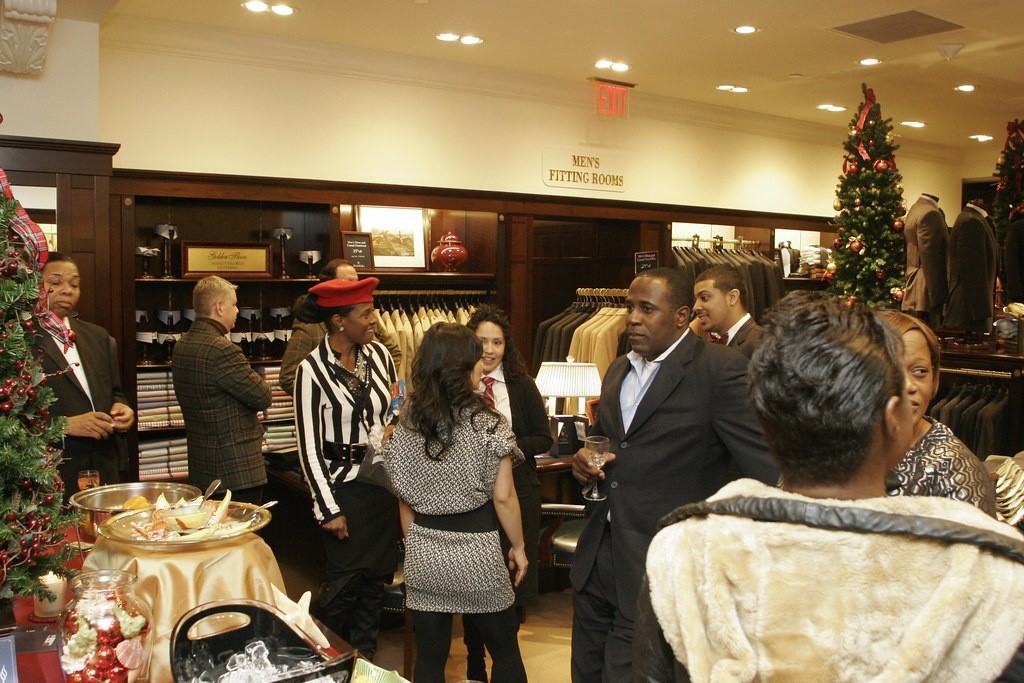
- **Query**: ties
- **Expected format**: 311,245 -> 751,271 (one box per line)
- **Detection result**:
482,377 -> 495,409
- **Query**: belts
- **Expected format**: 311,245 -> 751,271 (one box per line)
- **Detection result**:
322,440 -> 369,465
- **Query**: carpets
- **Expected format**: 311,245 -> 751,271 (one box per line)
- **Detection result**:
376,289 -> 480,316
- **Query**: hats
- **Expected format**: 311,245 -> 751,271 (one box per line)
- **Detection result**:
308,276 -> 379,307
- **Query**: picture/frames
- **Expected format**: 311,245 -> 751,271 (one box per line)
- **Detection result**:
586,400 -> 600,426
352,203 -> 431,272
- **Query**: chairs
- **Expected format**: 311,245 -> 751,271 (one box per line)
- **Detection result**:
537,504 -> 587,597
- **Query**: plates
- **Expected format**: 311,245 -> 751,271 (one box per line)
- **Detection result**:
97,500 -> 271,551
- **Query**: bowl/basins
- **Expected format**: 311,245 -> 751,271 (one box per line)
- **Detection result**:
163,502 -> 216,531
70,483 -> 200,541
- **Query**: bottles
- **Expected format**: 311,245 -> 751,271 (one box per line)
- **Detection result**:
57,569 -> 154,683
431,232 -> 469,272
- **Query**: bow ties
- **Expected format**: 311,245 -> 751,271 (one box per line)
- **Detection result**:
709,332 -> 730,347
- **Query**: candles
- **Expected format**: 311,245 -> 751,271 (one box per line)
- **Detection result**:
35,570 -> 64,614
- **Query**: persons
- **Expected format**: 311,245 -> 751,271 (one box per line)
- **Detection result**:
870,306 -> 996,519
637,291 -> 1024,683
946,197 -> 1002,330
22,249 -> 136,503
460,302 -> 556,683
293,275 -> 400,659
898,193 -> 951,315
385,322 -> 529,683
776,240 -> 800,278
568,267 -> 780,683
278,257 -> 404,397
168,278 -> 272,504
691,267 -> 777,359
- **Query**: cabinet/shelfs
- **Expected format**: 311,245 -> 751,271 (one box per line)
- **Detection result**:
110,167 -> 513,487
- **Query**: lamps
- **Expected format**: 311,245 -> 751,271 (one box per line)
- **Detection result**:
535,356 -> 602,415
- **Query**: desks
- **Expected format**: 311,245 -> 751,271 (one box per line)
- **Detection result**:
534,451 -> 577,504
79,534 -> 287,683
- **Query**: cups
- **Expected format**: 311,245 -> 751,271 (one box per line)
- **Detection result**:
78,470 -> 100,491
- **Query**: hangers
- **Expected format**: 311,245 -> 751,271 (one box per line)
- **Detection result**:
571,288 -> 632,310
671,237 -> 760,256
951,366 -> 1011,396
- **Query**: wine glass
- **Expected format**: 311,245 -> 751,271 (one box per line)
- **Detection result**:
581,436 -> 610,500
